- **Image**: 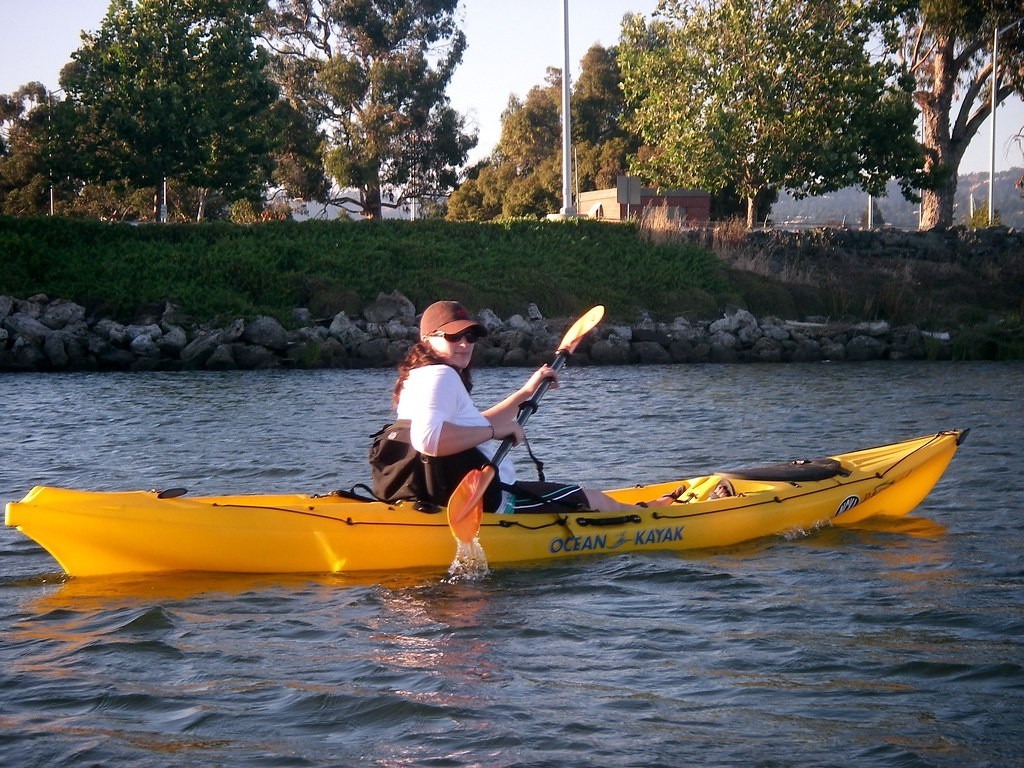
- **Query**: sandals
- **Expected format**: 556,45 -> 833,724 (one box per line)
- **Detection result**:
635,485 -> 687,507
710,478 -> 736,499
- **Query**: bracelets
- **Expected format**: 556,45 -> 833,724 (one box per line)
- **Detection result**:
488,425 -> 494,440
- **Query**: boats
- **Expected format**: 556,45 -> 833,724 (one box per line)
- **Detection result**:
5,428 -> 970,578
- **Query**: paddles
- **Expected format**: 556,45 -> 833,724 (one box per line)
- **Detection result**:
444,302 -> 606,547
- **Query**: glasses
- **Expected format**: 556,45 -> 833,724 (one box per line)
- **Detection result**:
426,329 -> 479,344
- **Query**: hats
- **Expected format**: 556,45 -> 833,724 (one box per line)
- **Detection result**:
420,301 -> 489,337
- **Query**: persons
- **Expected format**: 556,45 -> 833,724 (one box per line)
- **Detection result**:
390,301 -> 736,514
